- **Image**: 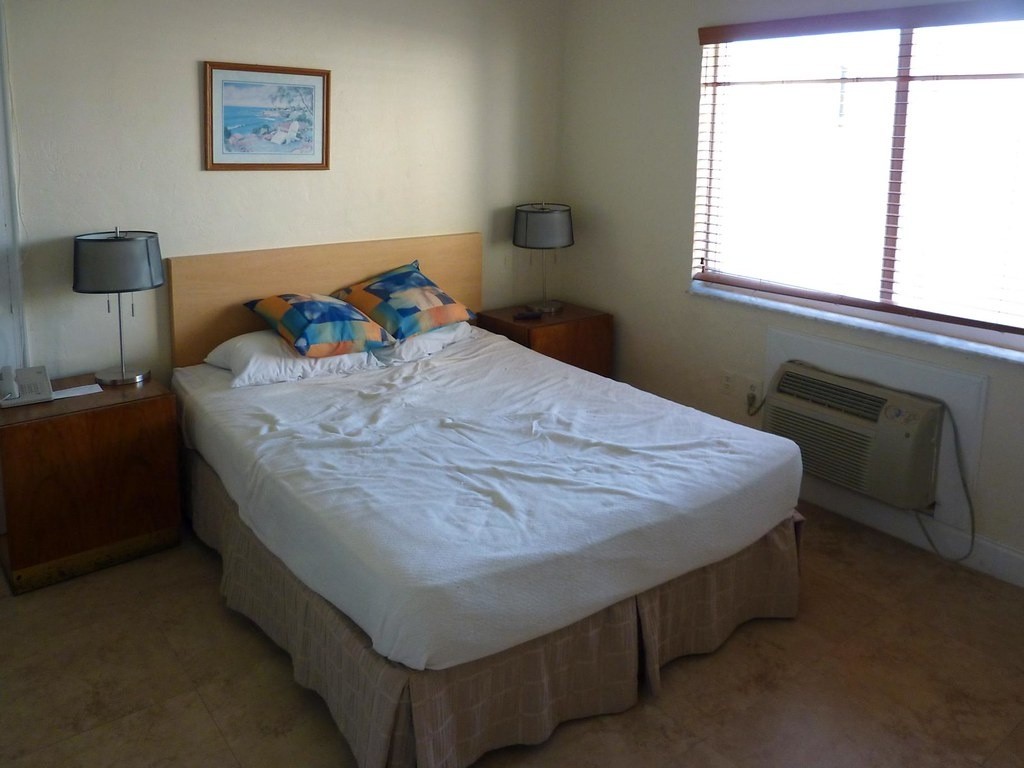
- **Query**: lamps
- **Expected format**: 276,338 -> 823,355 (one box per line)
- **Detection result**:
512,199 -> 575,313
72,226 -> 166,387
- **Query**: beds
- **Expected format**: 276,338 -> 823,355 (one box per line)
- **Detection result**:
155,231 -> 807,768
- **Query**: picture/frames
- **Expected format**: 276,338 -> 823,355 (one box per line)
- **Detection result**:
203,60 -> 332,171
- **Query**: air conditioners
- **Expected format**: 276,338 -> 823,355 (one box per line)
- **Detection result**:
762,359 -> 944,517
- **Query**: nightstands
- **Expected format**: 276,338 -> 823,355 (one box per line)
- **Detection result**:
0,373 -> 187,598
476,300 -> 614,379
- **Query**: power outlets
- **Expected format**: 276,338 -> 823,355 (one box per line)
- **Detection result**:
741,373 -> 764,410
719,368 -> 739,398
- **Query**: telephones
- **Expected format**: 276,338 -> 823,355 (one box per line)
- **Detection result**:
0,366 -> 53,409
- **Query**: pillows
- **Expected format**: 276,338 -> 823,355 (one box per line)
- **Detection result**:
372,321 -> 474,367
203,328 -> 388,389
243,292 -> 397,358
329,259 -> 477,344
